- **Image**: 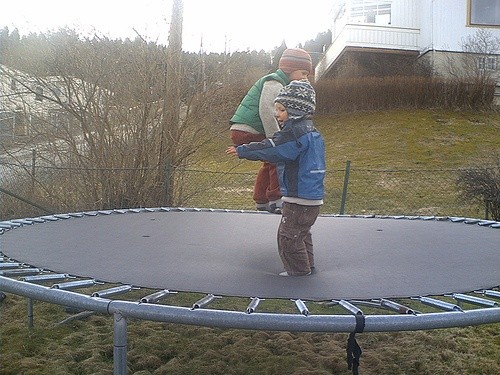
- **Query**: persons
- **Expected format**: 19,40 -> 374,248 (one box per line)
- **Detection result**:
228,47 -> 313,212
224,78 -> 326,277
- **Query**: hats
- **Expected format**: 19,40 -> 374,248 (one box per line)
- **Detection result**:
278,47 -> 312,74
273,78 -> 317,123
